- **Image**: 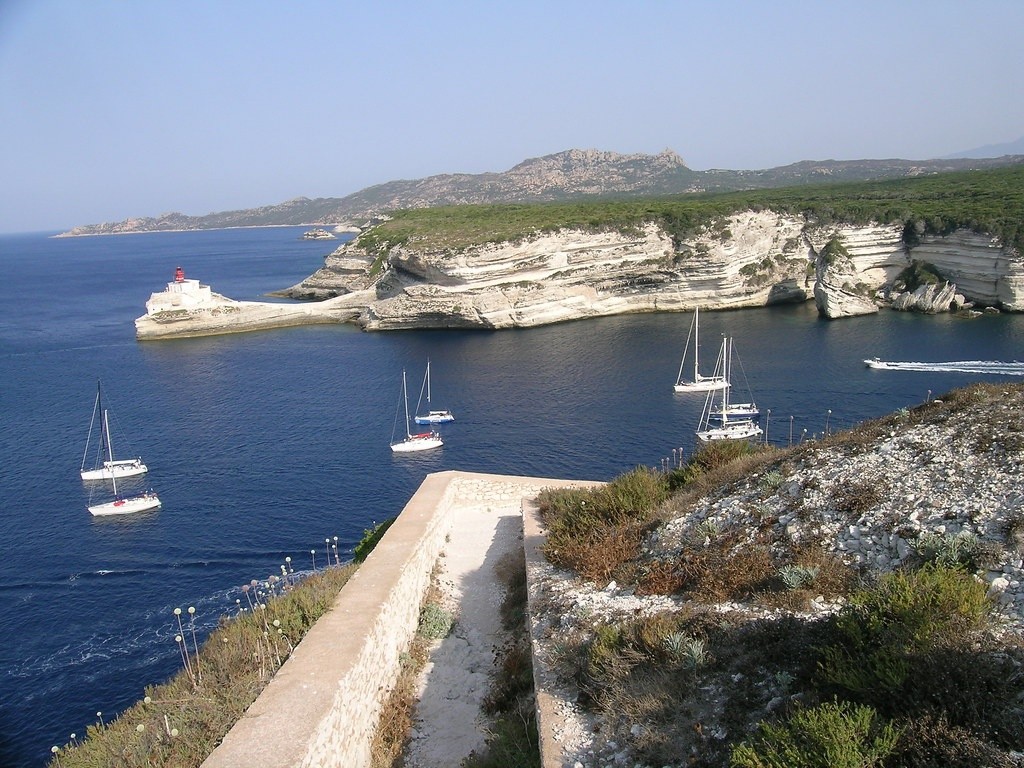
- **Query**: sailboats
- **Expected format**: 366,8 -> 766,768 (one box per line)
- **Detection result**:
413,357 -> 456,425
80,379 -> 148,480
695,332 -> 763,440
705,334 -> 761,423
390,366 -> 443,452
674,299 -> 732,393
87,411 -> 161,517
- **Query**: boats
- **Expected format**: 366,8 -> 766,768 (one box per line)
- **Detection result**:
863,357 -> 896,370
